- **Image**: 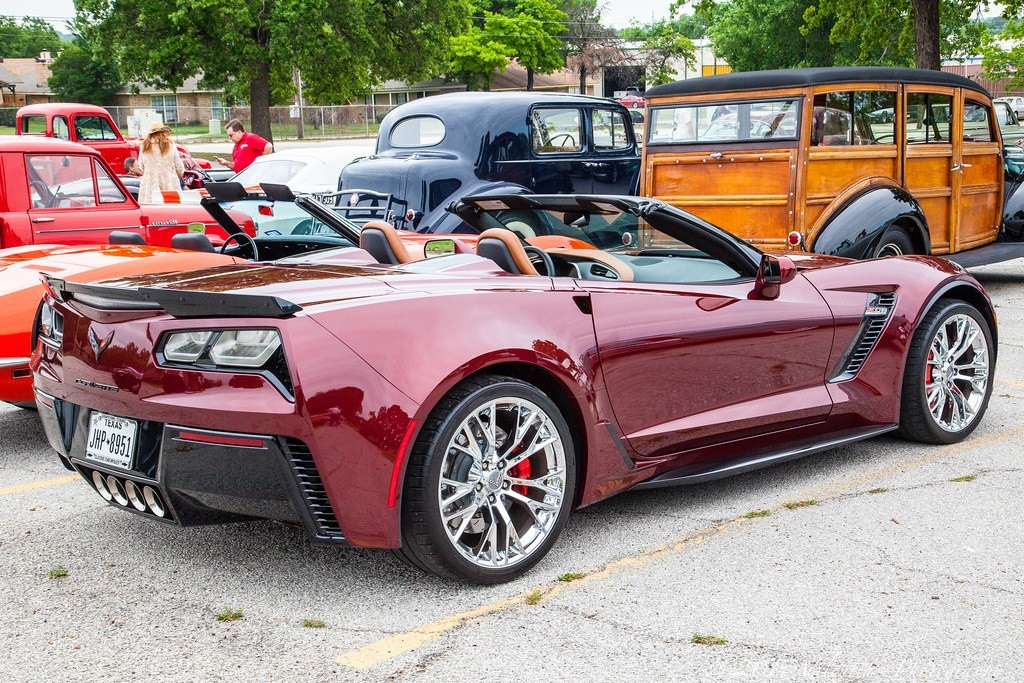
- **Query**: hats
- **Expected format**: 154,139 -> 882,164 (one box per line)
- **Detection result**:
144,121 -> 172,136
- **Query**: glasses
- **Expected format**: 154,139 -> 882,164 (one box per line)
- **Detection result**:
229,131 -> 239,138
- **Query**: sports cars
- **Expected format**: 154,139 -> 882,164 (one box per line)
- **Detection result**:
0,65 -> 1023,413
28,193 -> 999,586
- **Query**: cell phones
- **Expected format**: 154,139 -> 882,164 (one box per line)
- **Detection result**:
213,155 -> 220,161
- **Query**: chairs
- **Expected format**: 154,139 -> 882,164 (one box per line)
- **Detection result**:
171,233 -> 215,252
108,231 -> 144,244
474,227 -> 540,275
204,181 -> 247,201
259,182 -> 295,201
359,221 -> 413,265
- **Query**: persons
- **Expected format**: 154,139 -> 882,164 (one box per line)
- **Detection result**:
138,122 -> 185,205
124,157 -> 143,177
215,118 -> 273,174
